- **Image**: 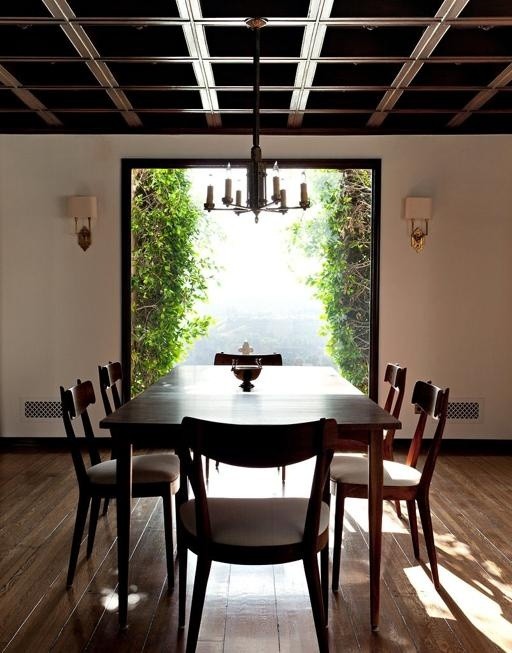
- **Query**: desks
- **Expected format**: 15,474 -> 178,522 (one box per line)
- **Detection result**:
98,363 -> 402,634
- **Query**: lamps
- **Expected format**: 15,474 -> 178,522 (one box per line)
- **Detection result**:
65,194 -> 98,252
403,196 -> 433,257
203,14 -> 312,224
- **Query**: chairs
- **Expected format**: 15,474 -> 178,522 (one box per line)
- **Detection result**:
205,352 -> 286,484
175,415 -> 338,653
59,360 -> 200,590
329,363 -> 450,594
